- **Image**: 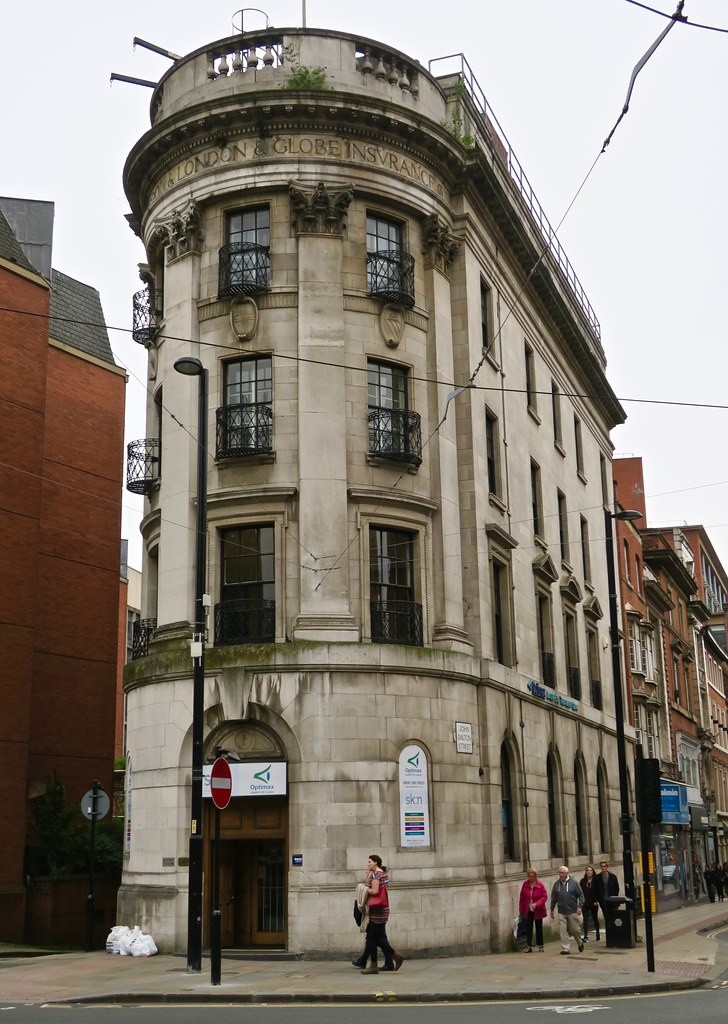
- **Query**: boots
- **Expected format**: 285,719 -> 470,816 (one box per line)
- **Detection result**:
392,951 -> 404,971
360,962 -> 378,974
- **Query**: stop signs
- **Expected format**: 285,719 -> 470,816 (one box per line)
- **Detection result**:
210,757 -> 232,809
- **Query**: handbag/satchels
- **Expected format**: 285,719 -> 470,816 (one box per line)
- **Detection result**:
106,925 -> 158,957
513,917 -> 527,939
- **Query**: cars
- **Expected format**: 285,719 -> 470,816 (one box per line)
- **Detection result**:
663,865 -> 680,884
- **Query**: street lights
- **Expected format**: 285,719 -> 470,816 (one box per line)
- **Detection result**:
604,510 -> 643,942
173,356 -> 211,973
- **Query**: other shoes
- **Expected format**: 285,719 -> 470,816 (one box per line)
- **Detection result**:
561,949 -> 570,954
579,943 -> 583,952
539,945 -> 544,952
379,964 -> 394,971
352,959 -> 367,969
525,947 -> 532,953
582,937 -> 588,942
596,934 -> 600,941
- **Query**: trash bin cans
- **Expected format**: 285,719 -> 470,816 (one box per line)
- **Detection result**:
604,896 -> 636,949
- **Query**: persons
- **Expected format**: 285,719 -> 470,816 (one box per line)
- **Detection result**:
351,854 -> 405,975
596,860 -> 619,921
579,864 -> 601,943
693,861 -> 728,903
519,867 -> 548,953
550,866 -> 584,955
